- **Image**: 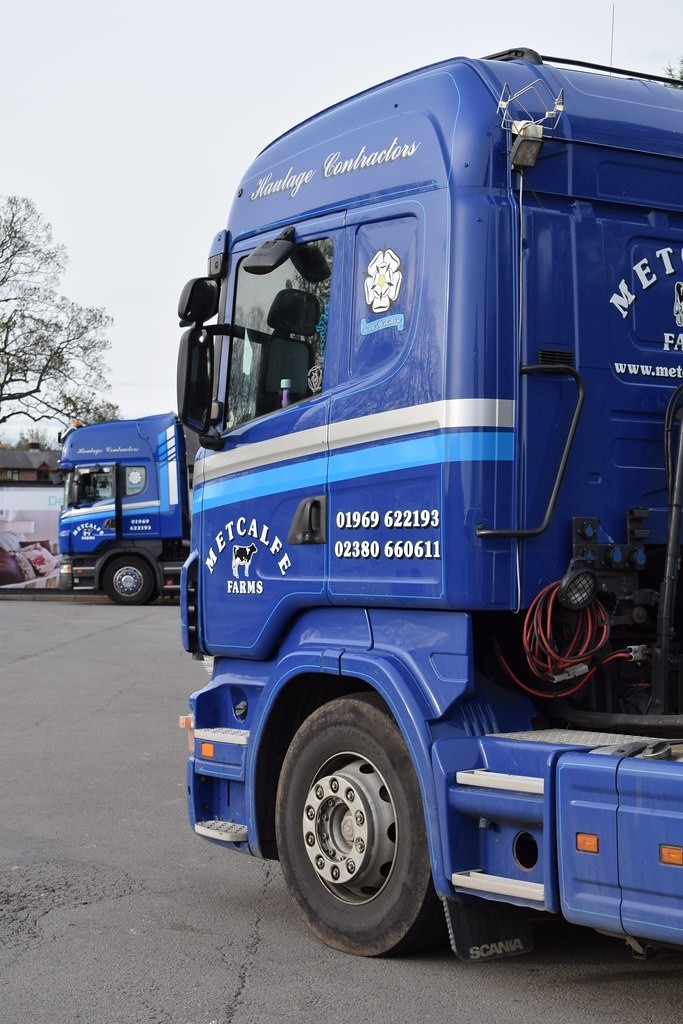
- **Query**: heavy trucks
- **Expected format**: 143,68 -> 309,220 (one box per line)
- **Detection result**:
175,44 -> 681,956
58,412 -> 190,604
